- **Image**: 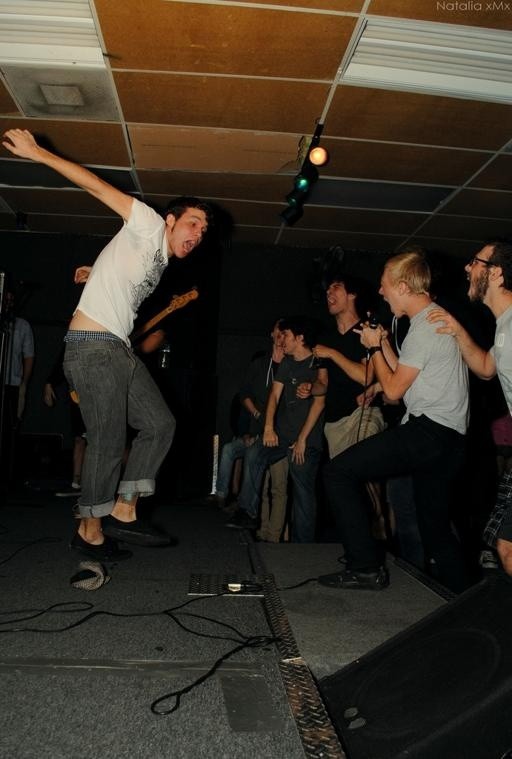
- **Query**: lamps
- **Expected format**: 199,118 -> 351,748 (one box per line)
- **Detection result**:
308,146 -> 330,169
285,189 -> 311,206
279,205 -> 304,226
293,166 -> 319,190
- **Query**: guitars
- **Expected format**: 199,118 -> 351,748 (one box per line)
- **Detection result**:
67,289 -> 199,404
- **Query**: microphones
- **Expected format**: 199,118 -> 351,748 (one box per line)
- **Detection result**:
365,315 -> 380,367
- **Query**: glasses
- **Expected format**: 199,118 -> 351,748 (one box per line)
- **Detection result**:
469,256 -> 488,266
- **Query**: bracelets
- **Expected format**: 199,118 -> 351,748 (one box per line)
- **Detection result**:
253,408 -> 260,418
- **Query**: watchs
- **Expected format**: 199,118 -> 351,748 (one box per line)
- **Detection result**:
367,345 -> 383,357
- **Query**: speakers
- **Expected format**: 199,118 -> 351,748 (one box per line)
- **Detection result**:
315,567 -> 512,759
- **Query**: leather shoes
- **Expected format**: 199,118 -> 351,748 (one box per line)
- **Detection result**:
318,568 -> 391,591
68,531 -> 132,563
102,513 -> 179,547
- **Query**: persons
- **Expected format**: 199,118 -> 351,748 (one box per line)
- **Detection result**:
36,264 -> 92,496
208,315 -> 288,548
426,237 -> 512,581
317,250 -> 468,590
255,455 -> 292,545
222,313 -> 330,544
0,127 -> 212,563
0,286 -> 36,510
310,268 -> 390,557
